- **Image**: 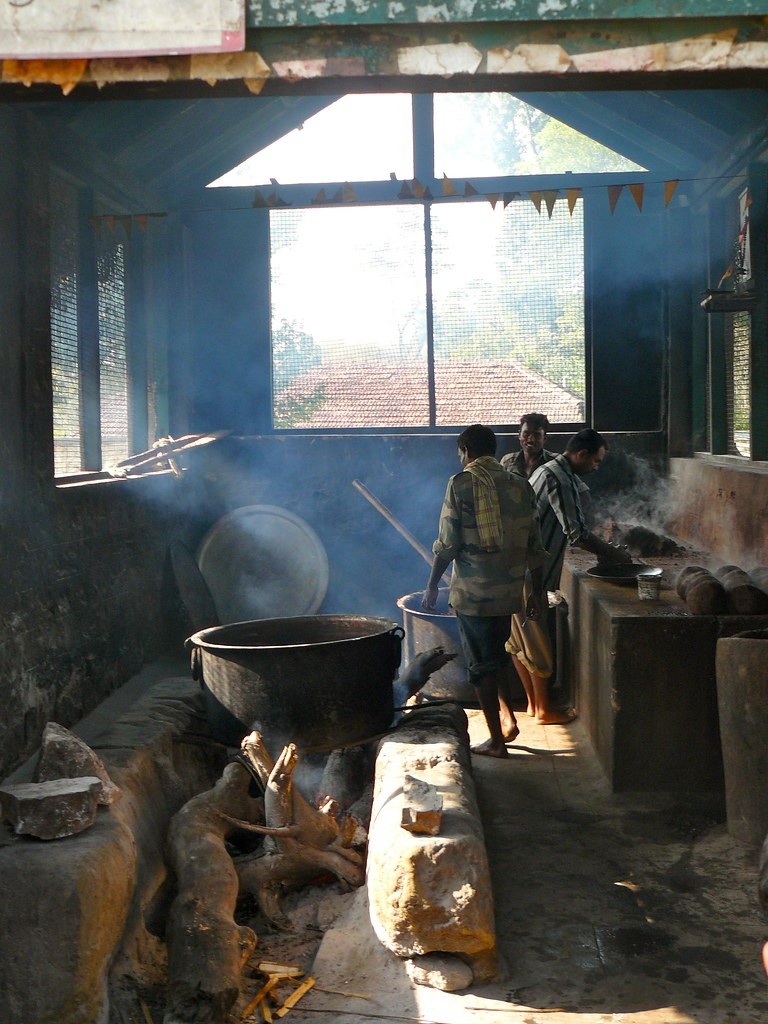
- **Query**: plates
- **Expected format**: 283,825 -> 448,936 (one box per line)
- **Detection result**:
586,564 -> 662,585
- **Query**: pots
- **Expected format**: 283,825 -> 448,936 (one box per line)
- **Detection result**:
397,588 -> 563,703
184,614 -> 399,756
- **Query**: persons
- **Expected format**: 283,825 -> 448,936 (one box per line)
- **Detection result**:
421,412 -> 633,759
